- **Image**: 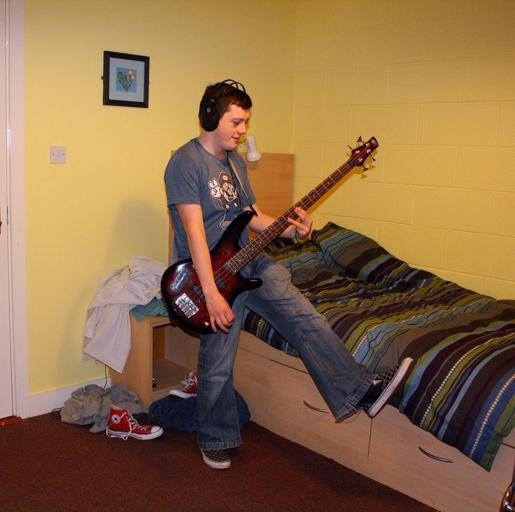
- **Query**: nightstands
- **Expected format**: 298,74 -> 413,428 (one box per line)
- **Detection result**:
108,313 -> 203,414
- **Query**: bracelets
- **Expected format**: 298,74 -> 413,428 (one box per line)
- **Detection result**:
292,230 -> 310,244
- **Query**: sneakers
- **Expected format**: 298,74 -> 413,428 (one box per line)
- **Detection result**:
107,407 -> 162,440
170,369 -> 201,398
357,355 -> 414,418
201,448 -> 231,469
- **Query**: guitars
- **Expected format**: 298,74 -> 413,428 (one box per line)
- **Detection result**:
161,135 -> 379,336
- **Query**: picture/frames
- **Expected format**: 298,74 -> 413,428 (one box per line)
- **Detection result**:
103,50 -> 150,108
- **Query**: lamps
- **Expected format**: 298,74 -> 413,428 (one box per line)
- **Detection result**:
246,135 -> 262,162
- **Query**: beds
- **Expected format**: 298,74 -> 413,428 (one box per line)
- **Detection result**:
170,152 -> 514,511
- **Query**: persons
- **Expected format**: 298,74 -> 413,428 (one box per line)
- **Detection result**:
208,172 -> 242,229
164,82 -> 414,470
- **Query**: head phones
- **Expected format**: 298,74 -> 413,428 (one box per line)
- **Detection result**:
200,80 -> 246,130
